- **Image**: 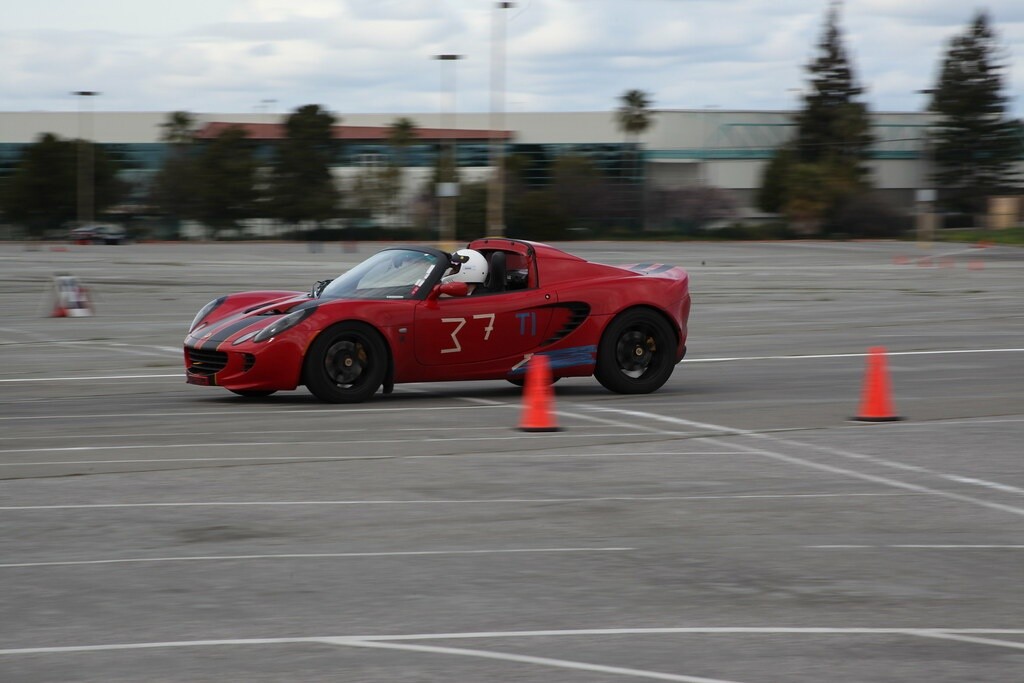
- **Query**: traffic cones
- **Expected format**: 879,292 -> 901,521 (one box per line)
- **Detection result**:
513,355 -> 565,432
848,346 -> 906,421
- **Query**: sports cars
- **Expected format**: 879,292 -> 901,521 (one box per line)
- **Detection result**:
182,236 -> 691,406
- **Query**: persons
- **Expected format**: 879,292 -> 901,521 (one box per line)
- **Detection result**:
440,249 -> 492,297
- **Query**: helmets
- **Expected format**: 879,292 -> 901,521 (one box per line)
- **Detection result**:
440,249 -> 488,284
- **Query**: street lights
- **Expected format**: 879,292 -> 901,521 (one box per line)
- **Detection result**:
433,54 -> 462,252
486,1 -> 517,241
70,90 -> 101,247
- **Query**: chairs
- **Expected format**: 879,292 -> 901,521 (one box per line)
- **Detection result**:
484,252 -> 508,295
